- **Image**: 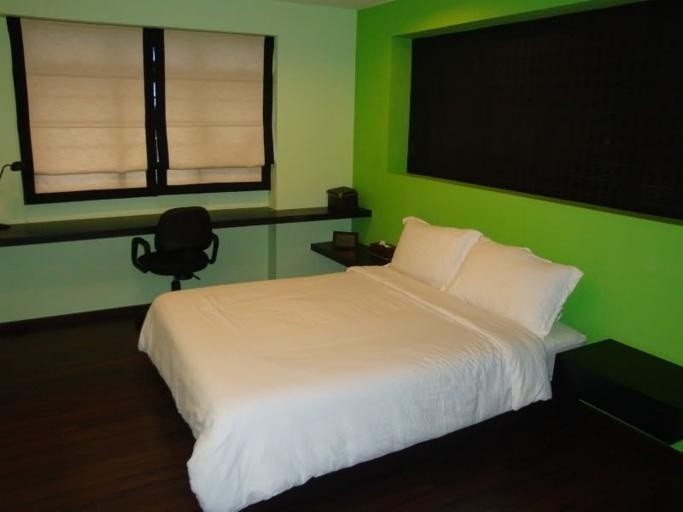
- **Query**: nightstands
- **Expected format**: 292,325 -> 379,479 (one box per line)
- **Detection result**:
554,338 -> 683,493
312,242 -> 396,268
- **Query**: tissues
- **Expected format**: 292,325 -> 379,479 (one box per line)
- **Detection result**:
370,240 -> 396,258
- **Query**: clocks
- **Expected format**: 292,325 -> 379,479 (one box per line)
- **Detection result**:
139,217 -> 587,511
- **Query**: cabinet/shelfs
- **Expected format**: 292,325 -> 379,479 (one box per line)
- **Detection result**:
0,206 -> 372,246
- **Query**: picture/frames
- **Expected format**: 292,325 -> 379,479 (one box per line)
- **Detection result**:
332,231 -> 359,246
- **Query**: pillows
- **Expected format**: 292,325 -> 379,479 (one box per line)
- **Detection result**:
385,217 -> 481,293
445,235 -> 584,340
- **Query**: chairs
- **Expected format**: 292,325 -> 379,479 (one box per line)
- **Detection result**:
131,205 -> 219,293
